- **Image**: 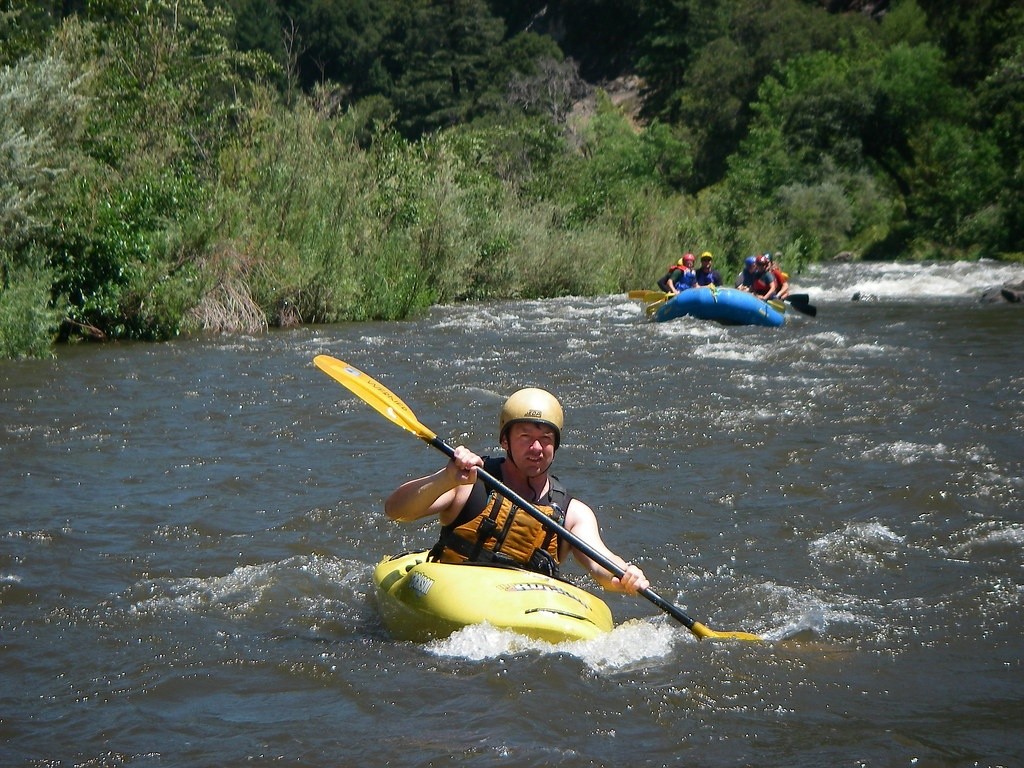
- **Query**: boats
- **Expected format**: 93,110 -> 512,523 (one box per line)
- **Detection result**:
373,544 -> 616,651
651,284 -> 788,330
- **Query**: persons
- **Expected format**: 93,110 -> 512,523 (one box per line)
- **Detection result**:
385,388 -> 650,597
657,251 -> 788,302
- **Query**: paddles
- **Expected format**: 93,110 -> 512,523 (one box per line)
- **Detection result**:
627,286 -> 677,316
312,353 -> 752,644
768,290 -> 818,318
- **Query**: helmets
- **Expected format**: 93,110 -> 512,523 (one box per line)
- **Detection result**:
677,258 -> 684,266
757,255 -> 769,264
499,388 -> 562,451
683,254 -> 695,261
744,256 -> 756,264
762,252 -> 772,261
701,251 -> 713,261
